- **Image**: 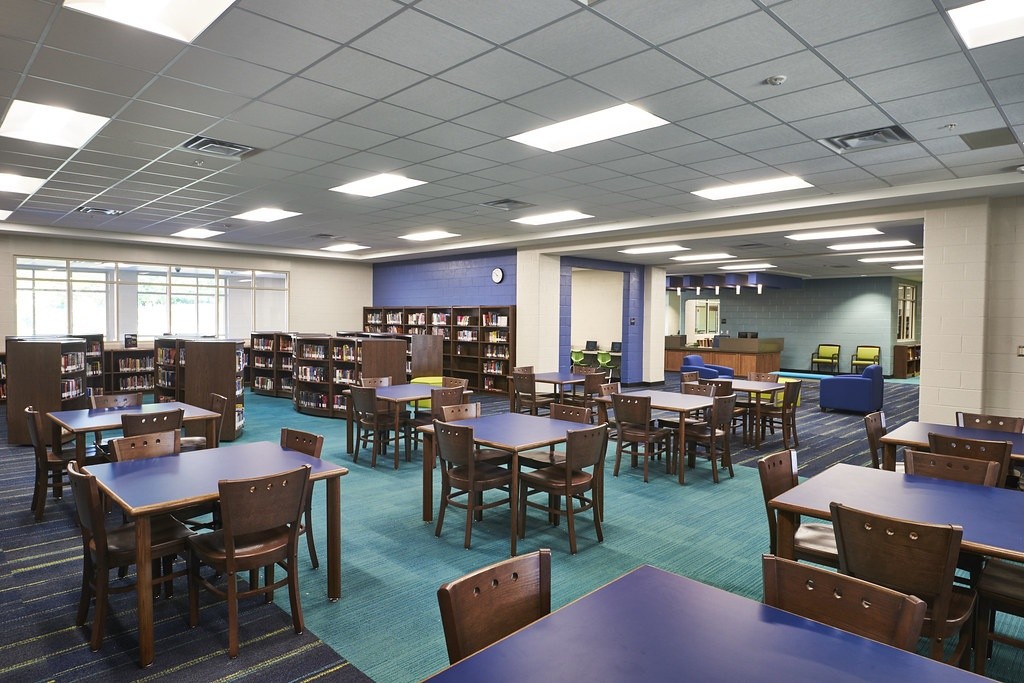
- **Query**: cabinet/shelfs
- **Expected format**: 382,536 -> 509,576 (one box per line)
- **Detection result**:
894,344 -> 920,379
0,302 -> 516,451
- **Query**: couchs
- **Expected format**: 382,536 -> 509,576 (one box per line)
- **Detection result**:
679,354 -> 735,391
819,364 -> 885,414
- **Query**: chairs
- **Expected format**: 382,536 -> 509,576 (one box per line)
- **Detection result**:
17,361 -> 1024,665
570,349 -> 590,370
850,344 -> 881,374
594,350 -> 617,382
809,343 -> 841,373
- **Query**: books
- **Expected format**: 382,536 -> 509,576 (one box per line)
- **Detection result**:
235,349 -> 248,430
0,362 -> 6,398
60,352 -> 84,400
254,335 -> 413,411
157,348 -> 185,387
364,312 -> 509,391
86,343 -> 103,397
118,357 -> 155,391
908,349 -> 920,360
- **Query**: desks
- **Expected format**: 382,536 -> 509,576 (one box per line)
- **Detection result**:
415,409 -> 611,555
880,418 -> 1024,488
420,562 -> 1000,683
45,398 -> 223,521
340,381 -> 470,471
505,371 -> 611,417
595,388 -> 714,486
767,459 -> 1024,649
80,438 -> 351,669
682,377 -> 785,450
